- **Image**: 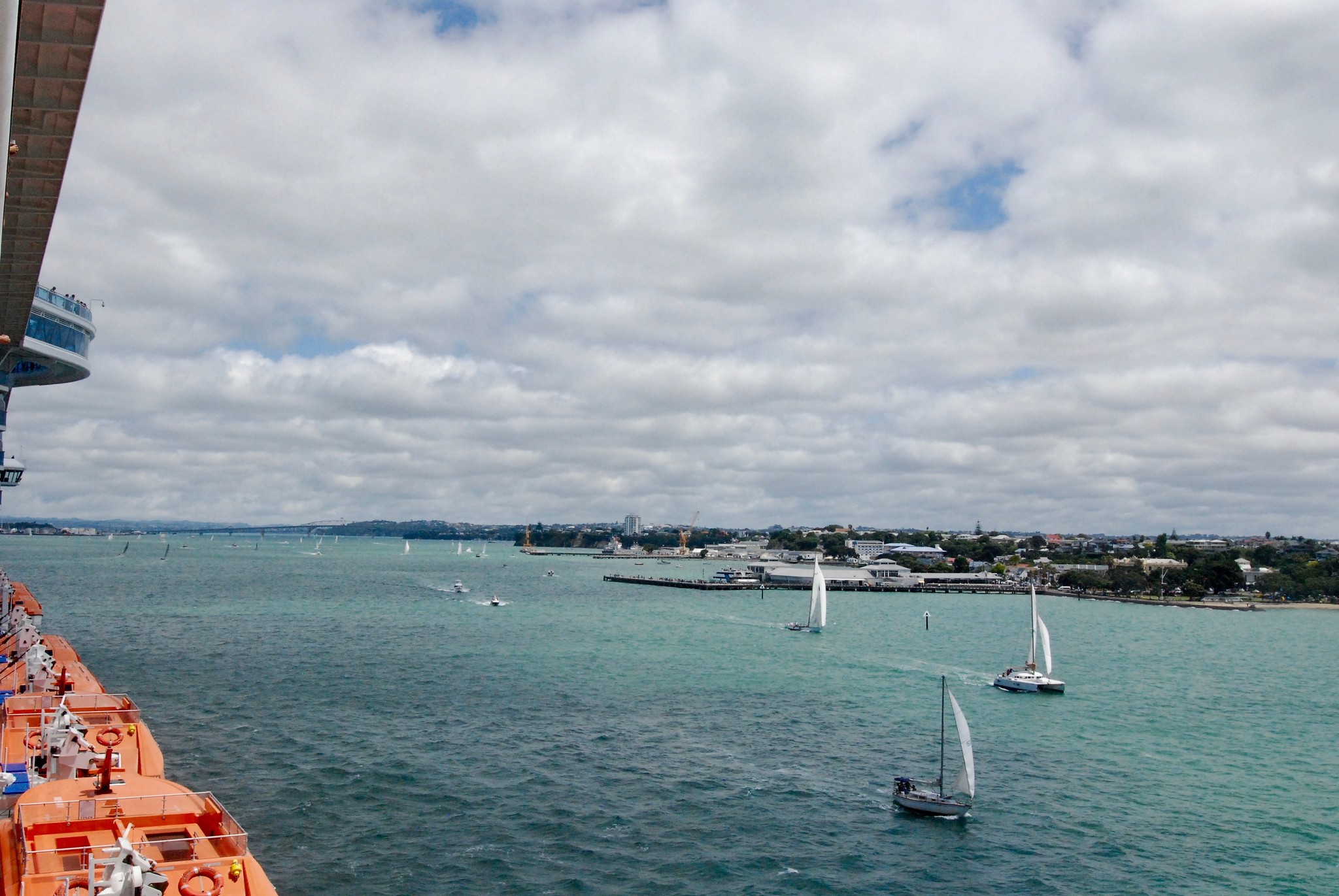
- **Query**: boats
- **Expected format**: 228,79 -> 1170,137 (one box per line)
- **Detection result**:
708,566 -> 760,583
629,540 -> 644,550
703,562 -> 711,565
0,725 -> 278,896
548,570 -> 554,577
490,595 -> 500,606
601,535 -> 622,554
0,569 -> 107,742
656,560 -> 670,565
0,666 -> 164,812
453,580 -> 463,590
675,564 -> 682,568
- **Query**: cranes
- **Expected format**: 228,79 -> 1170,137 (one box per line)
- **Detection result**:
522,519 -> 532,551
678,510 -> 700,554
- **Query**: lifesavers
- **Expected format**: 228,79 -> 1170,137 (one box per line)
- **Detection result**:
56,878 -> 102,896
179,866 -> 224,896
24,729 -> 48,749
98,727 -> 124,746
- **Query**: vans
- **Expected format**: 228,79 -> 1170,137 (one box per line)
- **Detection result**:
1058,586 -> 1071,592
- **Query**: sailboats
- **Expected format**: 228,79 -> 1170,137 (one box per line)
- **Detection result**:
784,551 -> 827,632
891,674 -> 977,819
23,525 -> 519,568
994,582 -> 1065,695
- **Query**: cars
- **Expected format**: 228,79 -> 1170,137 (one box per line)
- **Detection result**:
992,574 -> 1056,589
1065,587 -> 1339,605
